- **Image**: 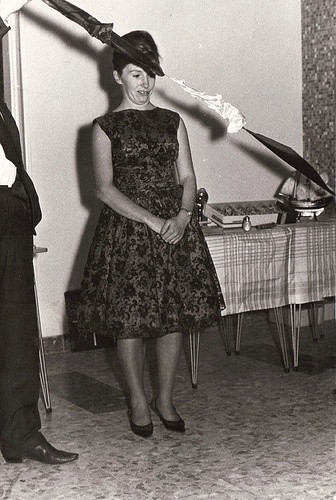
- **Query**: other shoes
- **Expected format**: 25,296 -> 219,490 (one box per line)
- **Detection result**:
152,396 -> 186,432
130,410 -> 154,438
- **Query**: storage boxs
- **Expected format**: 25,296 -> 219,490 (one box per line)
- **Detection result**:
203,199 -> 286,228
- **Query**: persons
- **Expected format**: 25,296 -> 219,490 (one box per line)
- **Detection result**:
75,30 -> 226,439
1,102 -> 79,463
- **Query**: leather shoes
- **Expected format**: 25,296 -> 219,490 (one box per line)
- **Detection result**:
6,438 -> 79,465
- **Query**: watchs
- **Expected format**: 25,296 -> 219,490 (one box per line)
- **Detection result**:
180,208 -> 192,216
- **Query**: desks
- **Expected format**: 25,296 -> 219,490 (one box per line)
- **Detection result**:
189,212 -> 336,389
